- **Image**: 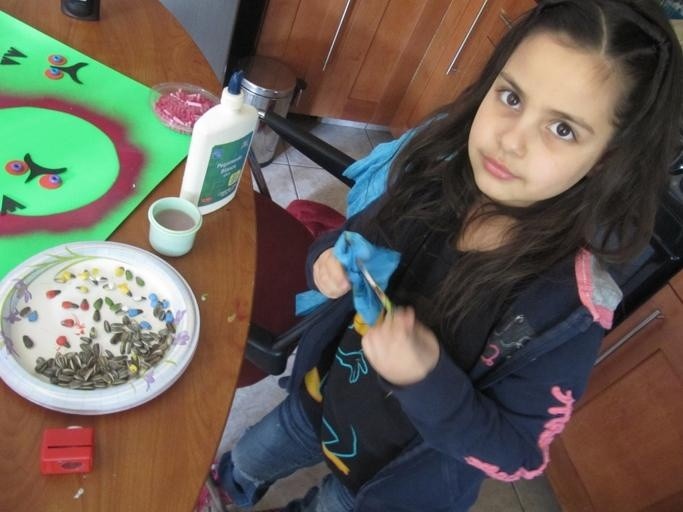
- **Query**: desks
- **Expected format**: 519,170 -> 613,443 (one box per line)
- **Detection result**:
0,0 -> 258,512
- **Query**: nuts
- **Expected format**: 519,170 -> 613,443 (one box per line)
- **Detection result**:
23,268 -> 175,390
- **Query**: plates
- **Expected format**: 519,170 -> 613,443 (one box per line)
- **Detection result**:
0,240 -> 201,420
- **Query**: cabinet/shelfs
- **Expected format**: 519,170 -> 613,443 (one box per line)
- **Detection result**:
256,1 -> 514,141
550,275 -> 680,512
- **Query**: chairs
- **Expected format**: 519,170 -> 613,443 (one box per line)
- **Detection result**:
242,102 -> 683,391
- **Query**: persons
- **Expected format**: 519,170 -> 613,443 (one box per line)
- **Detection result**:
198,0 -> 683,511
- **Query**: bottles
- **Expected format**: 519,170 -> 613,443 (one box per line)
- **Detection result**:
182,70 -> 260,217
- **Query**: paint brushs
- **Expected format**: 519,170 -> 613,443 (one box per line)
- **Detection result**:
355,258 -> 393,313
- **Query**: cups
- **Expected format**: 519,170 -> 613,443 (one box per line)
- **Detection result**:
146,195 -> 202,260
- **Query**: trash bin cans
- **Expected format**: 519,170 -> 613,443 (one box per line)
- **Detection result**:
239,56 -> 308,168
